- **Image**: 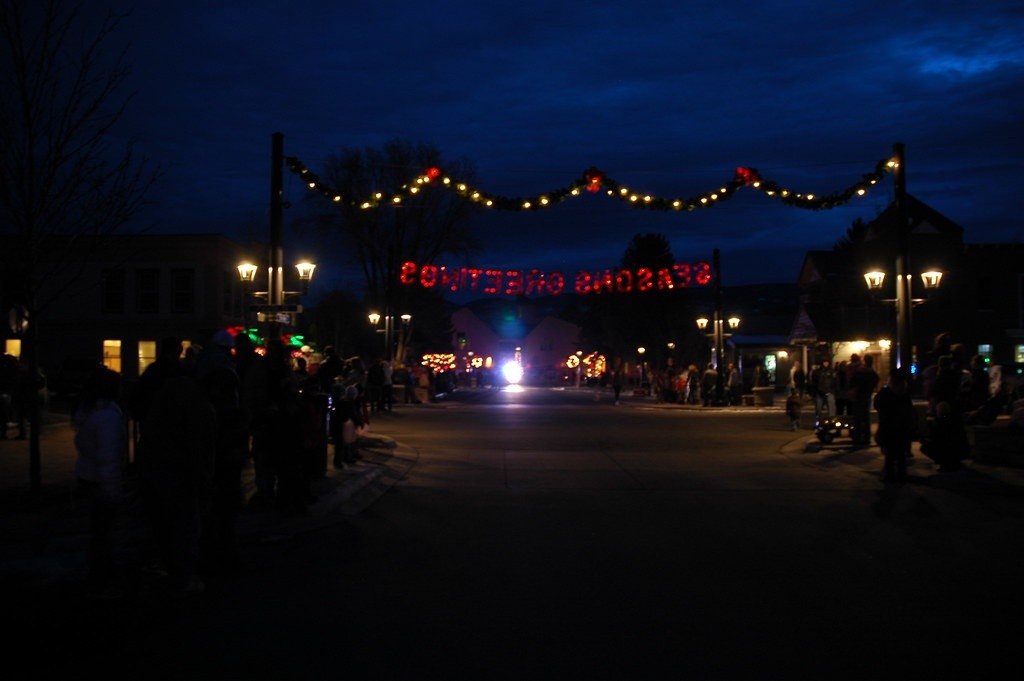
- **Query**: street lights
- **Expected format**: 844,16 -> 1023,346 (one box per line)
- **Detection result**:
864,269 -> 944,398
697,317 -> 740,396
366,312 -> 412,367
238,256 -> 317,342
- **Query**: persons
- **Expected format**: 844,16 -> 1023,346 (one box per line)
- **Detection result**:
0,334 -> 456,602
600,333 -> 1024,484
